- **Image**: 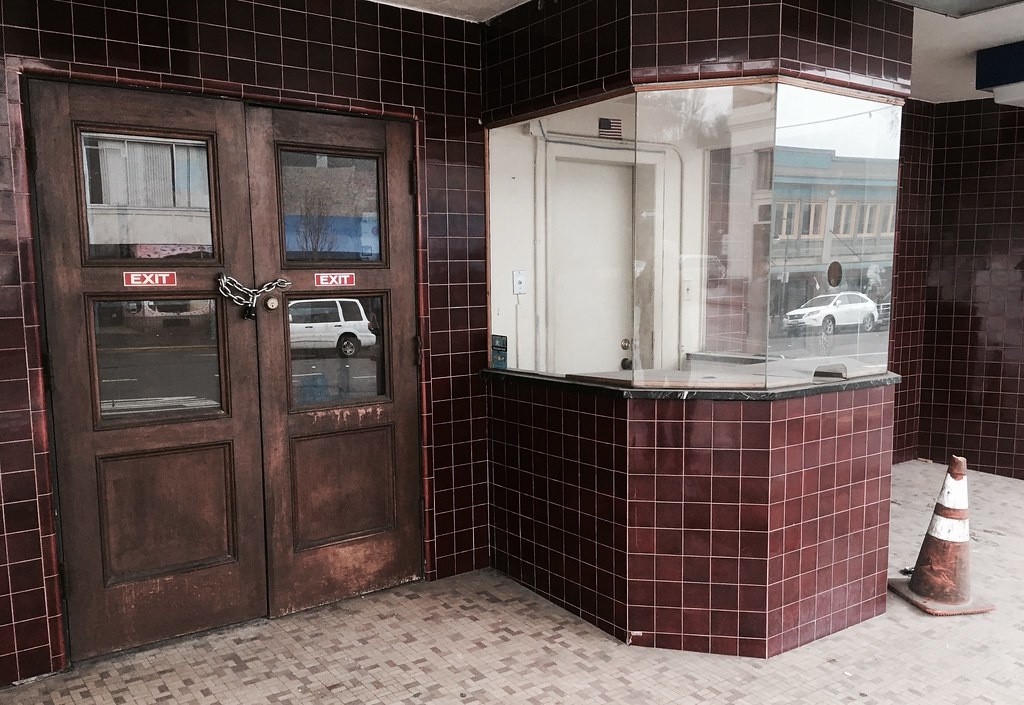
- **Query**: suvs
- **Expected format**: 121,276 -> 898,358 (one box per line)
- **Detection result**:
781,290 -> 879,337
877,290 -> 891,327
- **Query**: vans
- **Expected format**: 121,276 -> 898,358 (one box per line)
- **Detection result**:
287,298 -> 376,359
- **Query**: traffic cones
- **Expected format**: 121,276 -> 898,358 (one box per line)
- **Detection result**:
887,454 -> 994,618
